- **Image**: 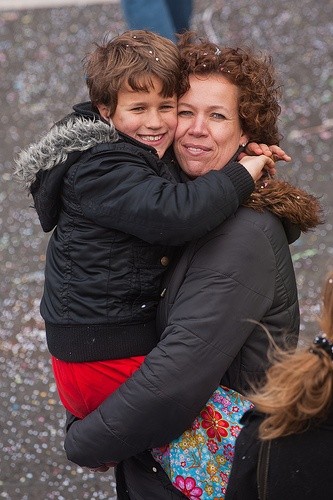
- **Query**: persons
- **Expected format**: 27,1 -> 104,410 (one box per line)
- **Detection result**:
64,30 -> 321,500
224,269 -> 332,500
12,28 -> 293,500
121,1 -> 195,46
